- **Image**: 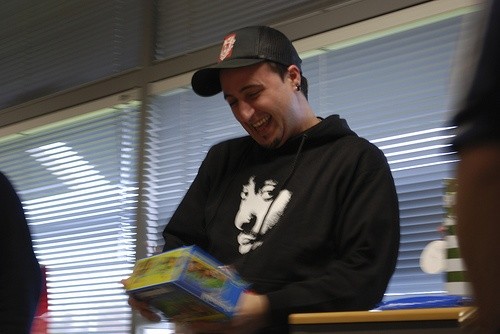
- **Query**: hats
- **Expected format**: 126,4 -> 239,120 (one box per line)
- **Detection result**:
191,25 -> 304,98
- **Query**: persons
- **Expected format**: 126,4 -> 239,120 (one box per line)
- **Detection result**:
0,172 -> 40,334
120,26 -> 401,334
439,1 -> 499,334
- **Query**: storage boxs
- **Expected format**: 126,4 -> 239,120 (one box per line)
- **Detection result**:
125,244 -> 250,324
